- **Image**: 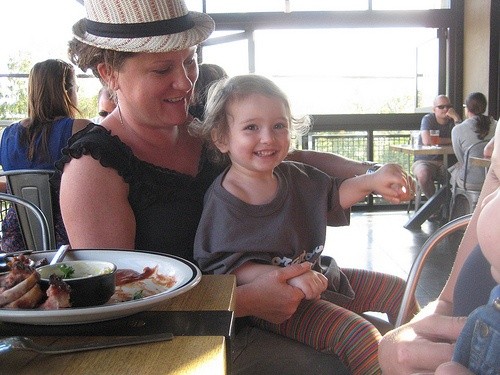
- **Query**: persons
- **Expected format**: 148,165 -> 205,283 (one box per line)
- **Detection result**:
435,191 -> 500,375
193,73 -> 422,375
450,91 -> 498,191
411,95 -> 464,223
89,63 -> 228,127
49,0 -> 395,375
0,59 -> 95,253
378,118 -> 500,374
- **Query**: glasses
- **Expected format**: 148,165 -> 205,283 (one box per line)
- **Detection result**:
436,104 -> 453,109
98,112 -> 108,117
463,104 -> 467,108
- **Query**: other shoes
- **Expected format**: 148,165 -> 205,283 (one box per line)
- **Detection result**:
428,210 -> 441,222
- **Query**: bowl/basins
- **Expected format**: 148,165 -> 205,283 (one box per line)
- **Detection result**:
36,260 -> 116,307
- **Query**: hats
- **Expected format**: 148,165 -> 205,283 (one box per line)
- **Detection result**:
71,0 -> 215,53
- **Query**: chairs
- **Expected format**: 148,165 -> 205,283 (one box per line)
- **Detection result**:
393,213 -> 475,332
448,140 -> 489,223
406,130 -> 442,213
0,192 -> 52,250
0,169 -> 61,251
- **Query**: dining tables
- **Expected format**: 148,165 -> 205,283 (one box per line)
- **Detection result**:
389,144 -> 454,230
0,273 -> 237,374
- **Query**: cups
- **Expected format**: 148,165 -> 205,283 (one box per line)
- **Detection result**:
429,130 -> 440,147
410,130 -> 422,149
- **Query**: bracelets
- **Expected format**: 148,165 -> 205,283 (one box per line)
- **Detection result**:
365,164 -> 383,174
454,120 -> 462,126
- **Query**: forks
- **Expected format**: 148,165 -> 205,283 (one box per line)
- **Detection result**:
0,332 -> 174,355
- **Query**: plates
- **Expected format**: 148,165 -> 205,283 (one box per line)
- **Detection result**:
0,248 -> 201,325
